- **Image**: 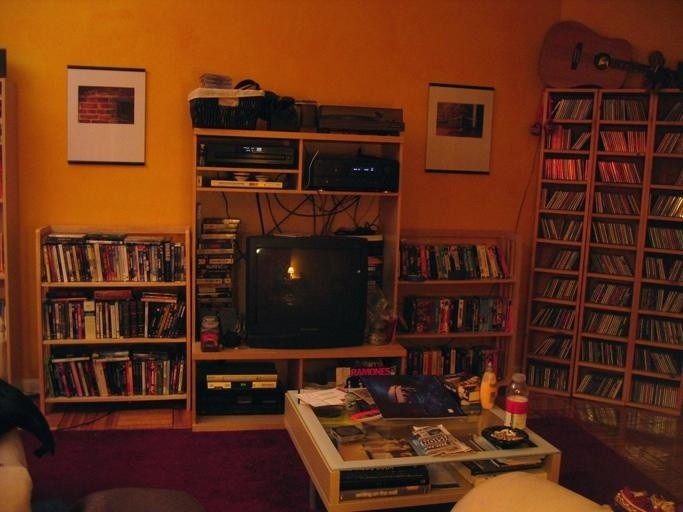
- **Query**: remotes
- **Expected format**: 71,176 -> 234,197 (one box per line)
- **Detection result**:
462,460 -> 481,476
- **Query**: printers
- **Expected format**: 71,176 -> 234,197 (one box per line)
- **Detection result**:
318,105 -> 406,135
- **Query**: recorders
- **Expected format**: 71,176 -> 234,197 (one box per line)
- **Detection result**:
312,153 -> 399,192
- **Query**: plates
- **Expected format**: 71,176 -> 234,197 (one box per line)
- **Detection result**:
483,425 -> 528,447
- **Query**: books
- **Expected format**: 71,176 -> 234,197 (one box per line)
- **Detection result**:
196,203 -> 242,313
409,344 -> 506,380
672,169 -> 683,186
540,216 -> 583,241
527,364 -> 567,393
547,96 -> 594,120
638,319 -> 683,345
340,466 -> 432,502
633,380 -> 679,408
448,430 -> 548,486
602,96 -> 647,121
530,306 -> 576,331
581,338 -> 625,368
647,226 -> 683,250
40,236 -> 186,282
656,132 -> 683,154
599,128 -> 646,154
399,242 -> 510,281
576,373 -> 623,401
640,287 -> 683,313
43,289 -> 186,341
648,192 -> 683,219
597,160 -> 642,184
542,278 -> 578,301
589,282 -> 631,307
634,346 -> 678,376
594,191 -> 641,216
44,350 -> 187,398
545,124 -> 592,152
551,248 -> 580,270
544,158 -> 590,181
583,311 -> 629,337
645,256 -> 683,281
409,296 -> 509,333
540,188 -> 585,211
659,98 -> 683,121
589,253 -> 633,276
359,375 -> 468,418
592,221 -> 634,246
533,336 -> 572,361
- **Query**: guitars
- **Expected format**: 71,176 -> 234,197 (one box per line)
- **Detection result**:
537,20 -> 683,90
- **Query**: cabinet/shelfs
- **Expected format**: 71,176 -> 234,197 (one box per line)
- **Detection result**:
396,235 -> 516,388
524,89 -> 658,406
191,125 -> 403,437
36,226 -> 191,417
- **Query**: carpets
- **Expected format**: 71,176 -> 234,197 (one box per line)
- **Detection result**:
21,416 -> 672,512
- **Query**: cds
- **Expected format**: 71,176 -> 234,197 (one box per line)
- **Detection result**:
201,74 -> 231,89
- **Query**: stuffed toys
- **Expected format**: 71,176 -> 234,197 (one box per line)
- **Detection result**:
197,315 -> 222,354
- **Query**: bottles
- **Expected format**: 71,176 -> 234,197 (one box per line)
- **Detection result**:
504,372 -> 528,429
480,361 -> 498,409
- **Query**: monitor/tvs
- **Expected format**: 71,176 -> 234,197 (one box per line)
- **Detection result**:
246,235 -> 368,349
